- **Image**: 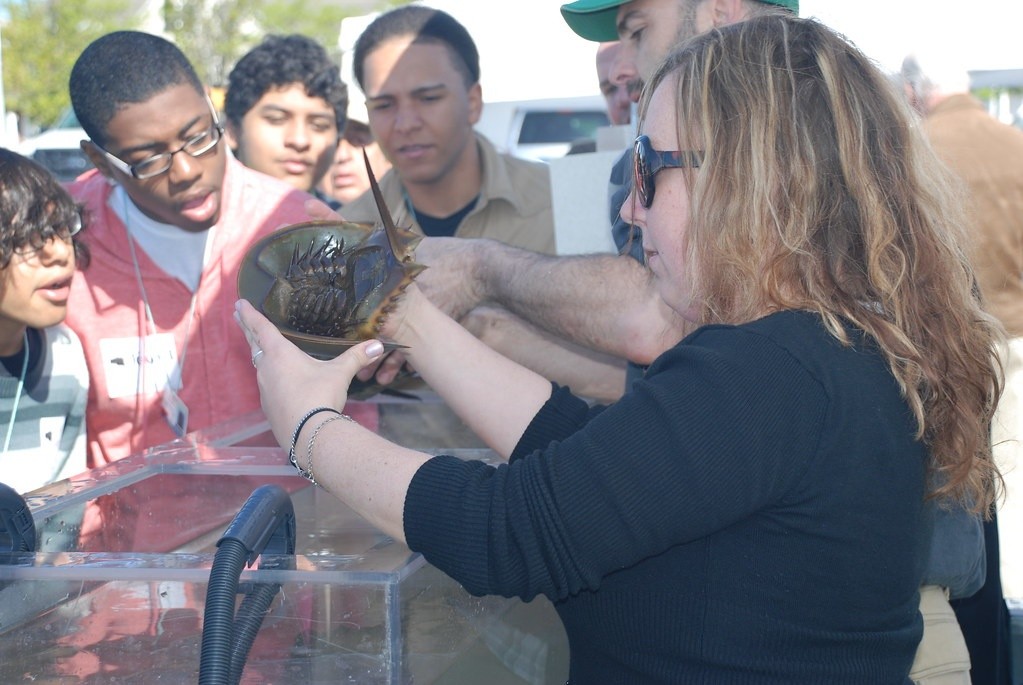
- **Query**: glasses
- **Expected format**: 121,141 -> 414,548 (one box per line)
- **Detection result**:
3,210 -> 82,254
90,95 -> 223,178
633,134 -> 705,206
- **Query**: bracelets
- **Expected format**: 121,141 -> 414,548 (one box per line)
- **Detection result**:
281,406 -> 354,492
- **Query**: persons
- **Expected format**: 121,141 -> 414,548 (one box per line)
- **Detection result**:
223,31 -> 349,219
558,0 -> 1012,685
593,36 -> 637,126
0,148 -> 89,553
54,28 -> 356,592
336,6 -> 556,452
309,59 -> 395,213
226,13 -> 1002,684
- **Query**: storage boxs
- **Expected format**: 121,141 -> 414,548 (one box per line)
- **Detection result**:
2,397 -> 568,684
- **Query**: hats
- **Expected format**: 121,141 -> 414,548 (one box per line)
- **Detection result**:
559,0 -> 798,41
